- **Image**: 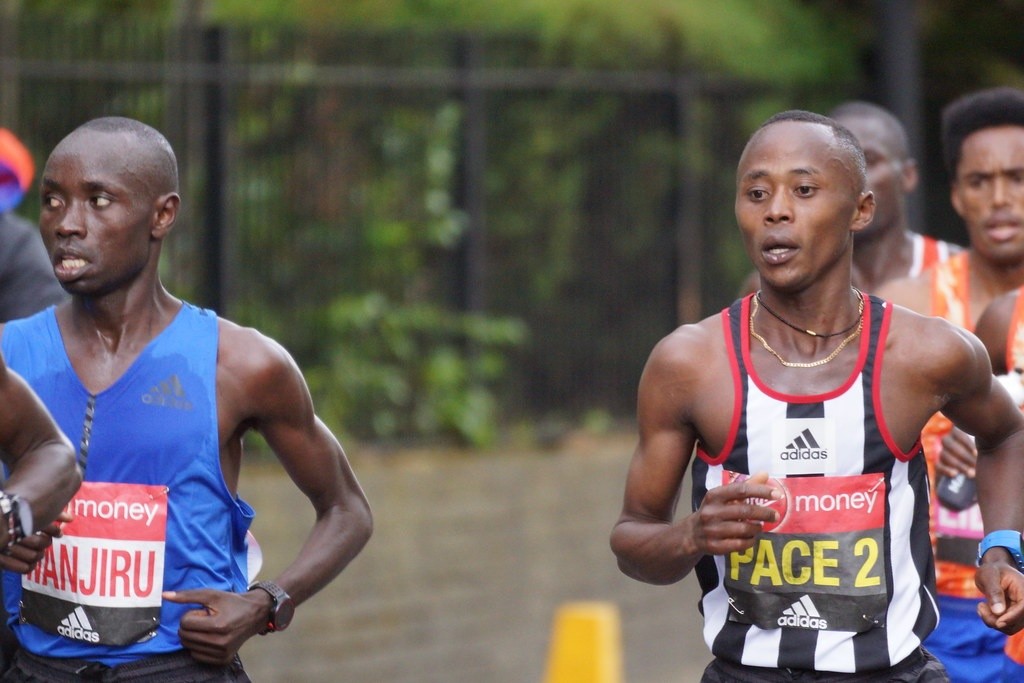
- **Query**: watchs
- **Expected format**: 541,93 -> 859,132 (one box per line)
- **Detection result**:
247,579 -> 295,636
0,490 -> 34,547
975,529 -> 1024,575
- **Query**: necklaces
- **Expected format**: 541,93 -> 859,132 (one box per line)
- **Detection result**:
749,285 -> 864,339
748,290 -> 862,368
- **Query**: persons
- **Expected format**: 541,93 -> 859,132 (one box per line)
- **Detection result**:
609,86 -> 1024,683
1,116 -> 374,683
